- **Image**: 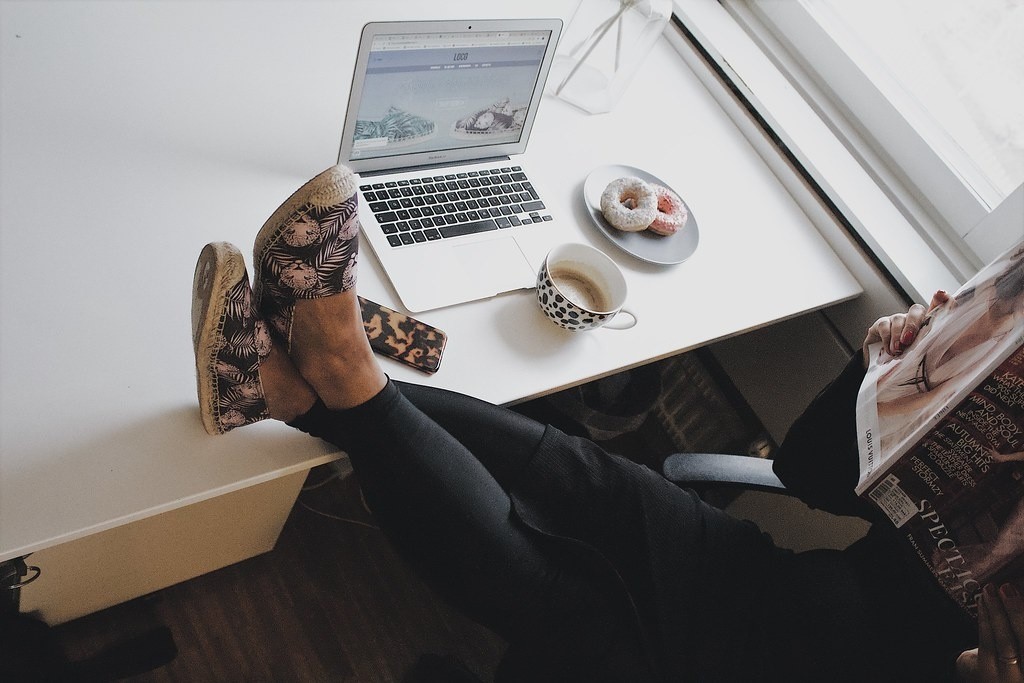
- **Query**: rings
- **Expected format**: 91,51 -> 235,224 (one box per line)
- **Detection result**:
999,658 -> 1018,665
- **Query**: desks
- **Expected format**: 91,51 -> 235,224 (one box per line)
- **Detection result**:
0,0 -> 865,628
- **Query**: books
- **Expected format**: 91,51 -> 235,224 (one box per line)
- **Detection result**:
852,235 -> 1024,625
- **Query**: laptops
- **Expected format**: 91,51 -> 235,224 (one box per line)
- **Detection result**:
338,18 -> 574,314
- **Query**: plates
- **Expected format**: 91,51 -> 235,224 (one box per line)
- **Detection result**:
584,163 -> 700,266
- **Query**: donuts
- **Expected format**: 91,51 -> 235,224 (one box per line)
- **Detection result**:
631,183 -> 688,236
601,177 -> 658,231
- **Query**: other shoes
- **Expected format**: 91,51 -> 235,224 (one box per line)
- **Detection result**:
191,240 -> 273,435
252,164 -> 361,359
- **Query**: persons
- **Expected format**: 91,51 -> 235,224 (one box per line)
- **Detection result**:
877,251 -> 1024,449
193,164 -> 1024,683
932,450 -> 1024,599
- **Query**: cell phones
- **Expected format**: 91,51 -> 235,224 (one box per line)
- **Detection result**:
356,295 -> 448,374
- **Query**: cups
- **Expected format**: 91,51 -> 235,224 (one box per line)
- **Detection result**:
534,242 -> 638,332
